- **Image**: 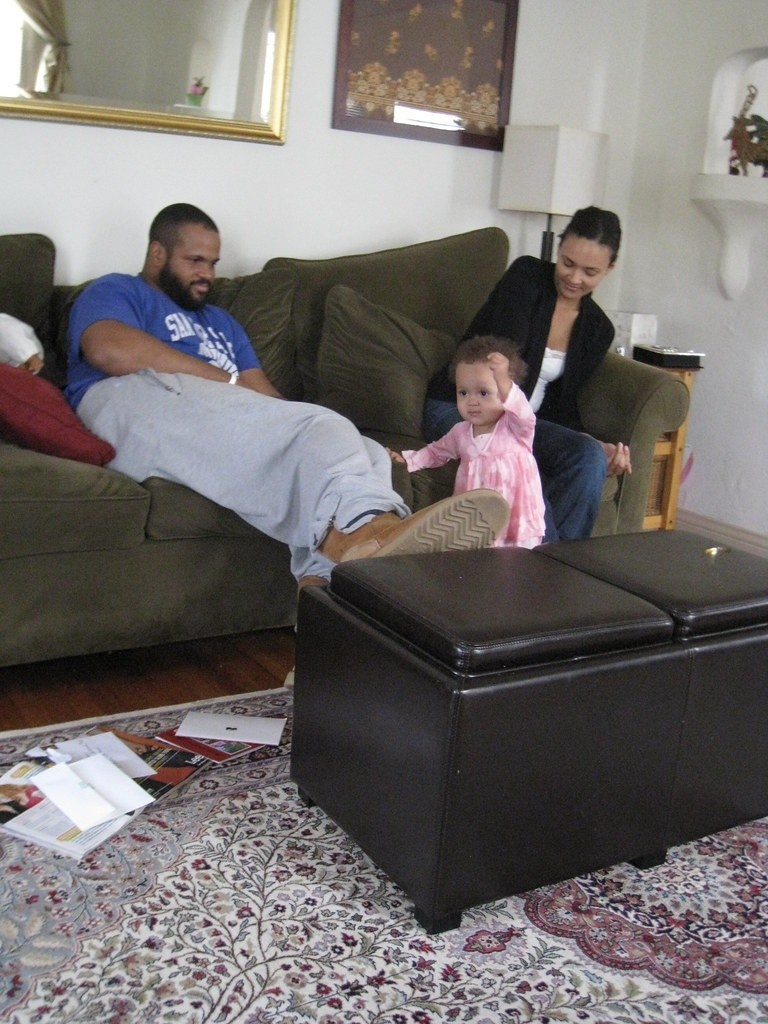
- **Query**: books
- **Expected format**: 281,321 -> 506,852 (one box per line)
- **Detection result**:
0,712 -> 289,863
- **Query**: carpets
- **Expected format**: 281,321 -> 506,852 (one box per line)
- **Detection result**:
0,690 -> 768,1024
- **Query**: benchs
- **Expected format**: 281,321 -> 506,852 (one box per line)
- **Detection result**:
282,527 -> 768,939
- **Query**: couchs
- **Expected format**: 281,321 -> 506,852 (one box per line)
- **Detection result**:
0,228 -> 689,674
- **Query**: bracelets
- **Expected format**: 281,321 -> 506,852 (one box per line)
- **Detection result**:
228,372 -> 237,385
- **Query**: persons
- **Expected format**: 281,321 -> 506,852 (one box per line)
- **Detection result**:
422,205 -> 632,543
384,335 -> 547,552
62,204 -> 511,692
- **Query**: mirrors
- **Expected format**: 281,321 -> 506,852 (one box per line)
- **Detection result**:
0,0 -> 297,147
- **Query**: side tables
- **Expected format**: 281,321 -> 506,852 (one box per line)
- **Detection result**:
640,359 -> 702,533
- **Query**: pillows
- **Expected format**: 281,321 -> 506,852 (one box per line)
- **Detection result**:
0,363 -> 117,469
0,230 -> 54,381
57,265 -> 303,416
316,283 -> 458,438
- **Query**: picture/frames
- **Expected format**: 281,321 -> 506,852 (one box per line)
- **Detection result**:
330,0 -> 519,153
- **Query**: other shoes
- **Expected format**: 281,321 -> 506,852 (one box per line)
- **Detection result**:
316,487 -> 510,565
295,576 -> 330,601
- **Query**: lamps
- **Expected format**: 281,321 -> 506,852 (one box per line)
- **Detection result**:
497,123 -> 602,262
